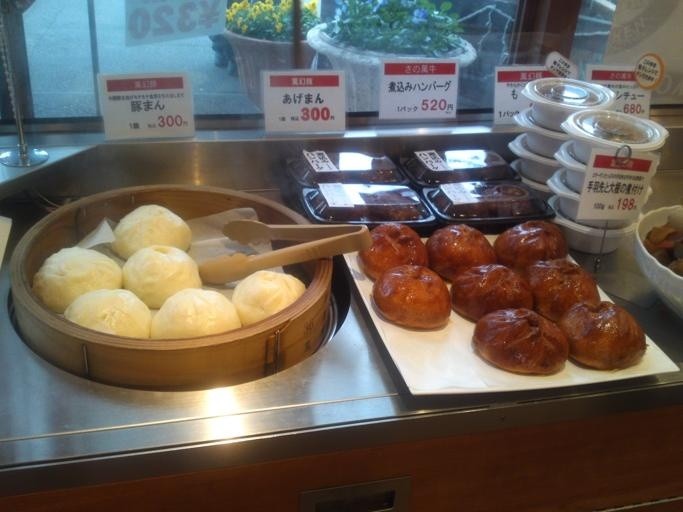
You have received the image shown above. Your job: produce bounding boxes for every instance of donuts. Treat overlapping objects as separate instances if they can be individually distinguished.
[298,152,540,220]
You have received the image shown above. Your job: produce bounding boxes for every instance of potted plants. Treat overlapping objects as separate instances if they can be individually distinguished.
[307,0,476,111]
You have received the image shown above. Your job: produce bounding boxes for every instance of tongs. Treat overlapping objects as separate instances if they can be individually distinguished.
[198,216,374,289]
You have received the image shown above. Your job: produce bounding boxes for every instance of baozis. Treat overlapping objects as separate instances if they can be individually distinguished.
[35,203,306,341]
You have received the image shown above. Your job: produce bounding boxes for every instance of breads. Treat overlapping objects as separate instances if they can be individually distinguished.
[357,222,645,375]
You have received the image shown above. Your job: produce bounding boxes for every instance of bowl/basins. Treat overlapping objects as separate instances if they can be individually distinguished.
[507,72,669,257]
[631,203,683,316]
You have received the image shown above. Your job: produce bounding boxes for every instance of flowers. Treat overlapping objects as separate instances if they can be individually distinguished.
[223,0,321,41]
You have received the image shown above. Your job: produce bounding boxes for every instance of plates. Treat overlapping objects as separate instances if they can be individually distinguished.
[337,228,681,395]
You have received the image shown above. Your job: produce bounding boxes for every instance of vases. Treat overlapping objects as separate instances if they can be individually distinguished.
[226,30,316,109]
